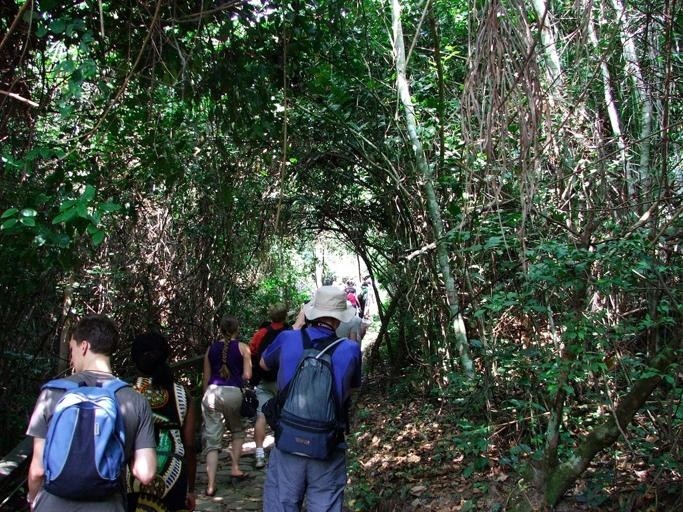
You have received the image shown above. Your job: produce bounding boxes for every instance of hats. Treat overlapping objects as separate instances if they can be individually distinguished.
[302,284,357,323]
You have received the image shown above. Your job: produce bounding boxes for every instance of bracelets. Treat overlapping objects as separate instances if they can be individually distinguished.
[26,494,33,503]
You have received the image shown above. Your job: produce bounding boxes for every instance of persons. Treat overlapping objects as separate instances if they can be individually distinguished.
[25,313,158,512]
[122,331,196,512]
[249,301,293,468]
[336,278,368,341]
[260,285,362,512]
[199,313,255,497]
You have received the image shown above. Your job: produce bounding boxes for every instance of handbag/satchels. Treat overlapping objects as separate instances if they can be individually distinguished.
[241,388,259,418]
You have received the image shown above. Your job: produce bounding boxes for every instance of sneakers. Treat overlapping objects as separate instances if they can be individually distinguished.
[255,456,266,468]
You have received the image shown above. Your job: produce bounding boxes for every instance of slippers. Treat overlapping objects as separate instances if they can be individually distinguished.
[228,470,249,480]
[206,484,218,497]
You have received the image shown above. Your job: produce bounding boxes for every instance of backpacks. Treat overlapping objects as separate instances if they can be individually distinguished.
[260,326,352,461]
[255,324,289,381]
[38,378,133,502]
